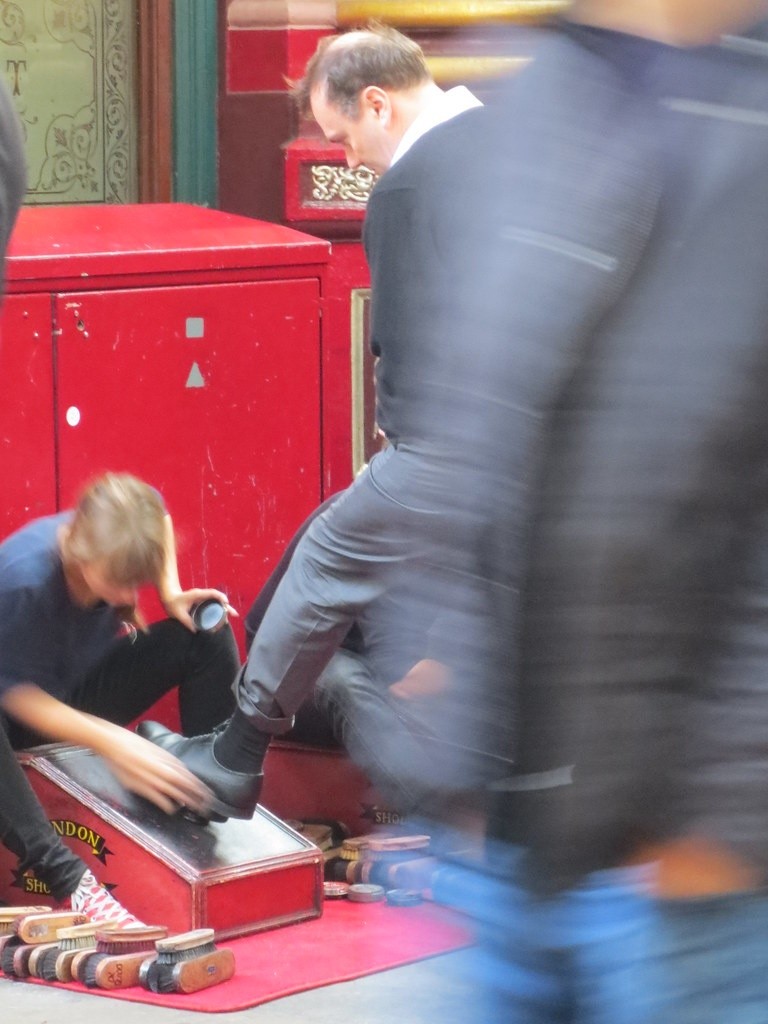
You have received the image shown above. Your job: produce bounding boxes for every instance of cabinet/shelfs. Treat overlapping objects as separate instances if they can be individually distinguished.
[0,200,387,741]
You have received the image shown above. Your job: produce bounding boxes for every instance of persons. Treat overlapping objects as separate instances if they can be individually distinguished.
[244,464,508,818]
[354,1,768,1022]
[1,469,246,936]
[131,22,508,820]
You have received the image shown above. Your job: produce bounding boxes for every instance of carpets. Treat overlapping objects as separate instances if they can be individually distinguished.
[0,897,481,1013]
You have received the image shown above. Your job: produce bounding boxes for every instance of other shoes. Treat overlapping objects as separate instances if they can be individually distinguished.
[68,874,144,932]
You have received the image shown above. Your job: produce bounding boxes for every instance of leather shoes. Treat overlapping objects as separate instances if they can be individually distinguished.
[134,719,263,819]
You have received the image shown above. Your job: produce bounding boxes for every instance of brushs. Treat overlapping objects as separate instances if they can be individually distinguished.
[316,832,437,888]
[0,905,237,995]
